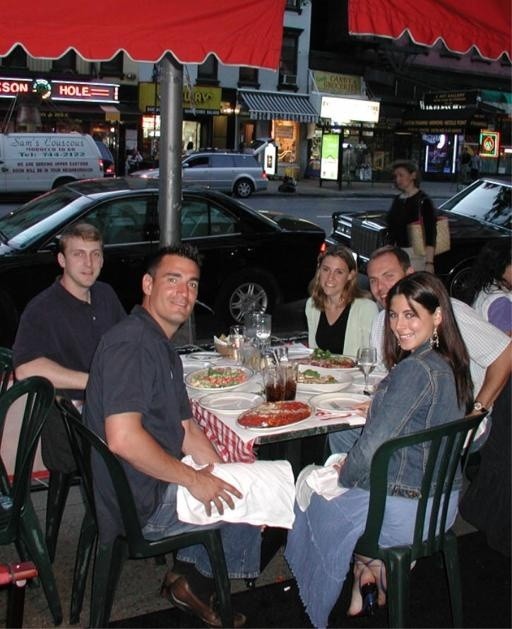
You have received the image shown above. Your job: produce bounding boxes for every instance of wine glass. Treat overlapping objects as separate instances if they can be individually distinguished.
[230,313,271,369]
[356,345,377,394]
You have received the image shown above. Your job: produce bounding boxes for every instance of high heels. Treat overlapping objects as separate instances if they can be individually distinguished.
[345,559,417,617]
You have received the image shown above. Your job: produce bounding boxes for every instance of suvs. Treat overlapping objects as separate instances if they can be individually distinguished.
[126,147,271,202]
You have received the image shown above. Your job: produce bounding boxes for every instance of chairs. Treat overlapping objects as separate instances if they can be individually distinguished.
[28,382,148,567]
[0,374,67,628]
[354,406,488,629]
[56,396,234,629]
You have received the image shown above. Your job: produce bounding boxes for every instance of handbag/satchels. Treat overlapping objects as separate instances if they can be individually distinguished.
[406,216,451,257]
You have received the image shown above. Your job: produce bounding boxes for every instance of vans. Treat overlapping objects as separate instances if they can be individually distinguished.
[0,130,117,198]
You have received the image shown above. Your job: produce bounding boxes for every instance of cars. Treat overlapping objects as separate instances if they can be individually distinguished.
[0,178,329,332]
[332,177,509,299]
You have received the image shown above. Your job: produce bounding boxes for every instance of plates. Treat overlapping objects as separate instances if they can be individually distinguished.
[236,401,312,432]
[185,365,253,392]
[307,355,360,372]
[297,364,354,394]
[308,393,372,413]
[199,392,264,414]
[288,347,314,360]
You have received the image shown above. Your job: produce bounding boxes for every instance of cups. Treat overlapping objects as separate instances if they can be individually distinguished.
[262,361,298,401]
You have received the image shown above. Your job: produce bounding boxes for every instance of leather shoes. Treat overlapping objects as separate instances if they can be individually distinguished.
[161,572,246,627]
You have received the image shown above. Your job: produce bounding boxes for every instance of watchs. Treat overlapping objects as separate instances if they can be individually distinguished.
[472,400,489,415]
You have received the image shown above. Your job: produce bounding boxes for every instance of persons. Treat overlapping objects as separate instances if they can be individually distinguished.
[81,242,264,629]
[283,270,475,618]
[13,222,127,478]
[472,238,512,338]
[365,244,511,455]
[305,246,379,362]
[388,159,438,271]
